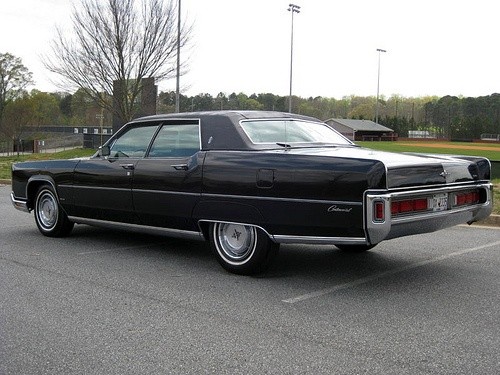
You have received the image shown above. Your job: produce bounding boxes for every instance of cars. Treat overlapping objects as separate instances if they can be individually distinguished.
[11,109,495,276]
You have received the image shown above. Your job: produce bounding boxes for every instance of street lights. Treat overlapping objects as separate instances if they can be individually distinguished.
[286,3,301,113]
[376,49,387,122]
